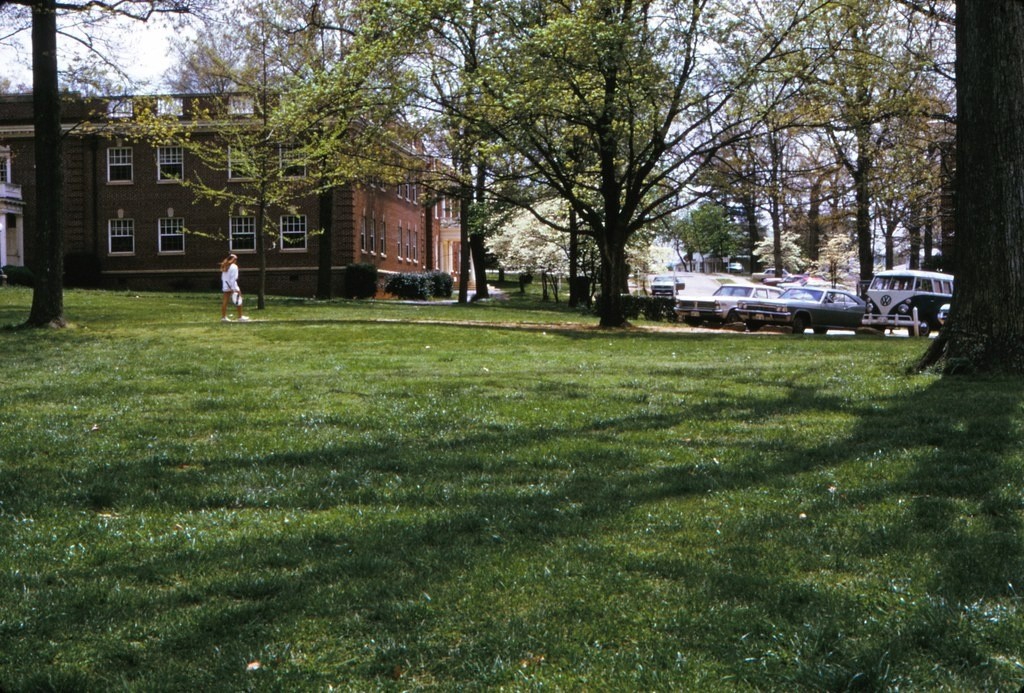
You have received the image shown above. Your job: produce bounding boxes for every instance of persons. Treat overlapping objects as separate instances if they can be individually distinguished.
[222,254,248,322]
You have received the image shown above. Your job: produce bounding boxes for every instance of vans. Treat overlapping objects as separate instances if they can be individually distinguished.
[861,268,954,337]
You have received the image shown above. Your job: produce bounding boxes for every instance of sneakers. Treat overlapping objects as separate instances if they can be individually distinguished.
[221,316,231,322]
[238,316,249,321]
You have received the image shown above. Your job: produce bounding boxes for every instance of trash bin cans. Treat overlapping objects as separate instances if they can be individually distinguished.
[567,276,590,306]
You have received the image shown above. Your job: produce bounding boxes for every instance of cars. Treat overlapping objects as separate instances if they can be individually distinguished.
[667,267,858,309]
[726,262,744,274]
[673,284,786,329]
[734,285,866,334]
[650,274,677,296]
